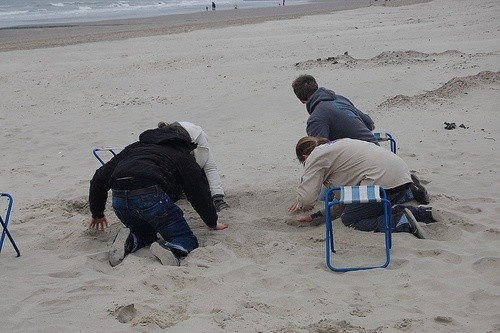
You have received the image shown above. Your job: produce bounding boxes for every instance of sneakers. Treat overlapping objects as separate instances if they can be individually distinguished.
[402,208,426,241]
[421,206,439,223]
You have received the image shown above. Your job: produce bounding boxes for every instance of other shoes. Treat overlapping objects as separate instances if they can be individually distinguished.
[409,182,429,206]
[212,196,231,213]
[411,173,420,184]
[149,242,180,267]
[108,227,131,267]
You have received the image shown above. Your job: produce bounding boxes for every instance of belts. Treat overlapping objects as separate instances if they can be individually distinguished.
[112,185,160,197]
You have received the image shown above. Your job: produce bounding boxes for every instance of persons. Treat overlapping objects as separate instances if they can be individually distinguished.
[289,135,438,239]
[155,121,230,212]
[89,121,227,267]
[292,74,430,206]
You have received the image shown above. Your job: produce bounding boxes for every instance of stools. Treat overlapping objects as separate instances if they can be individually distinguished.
[324,186,392,272]
[374,132,397,154]
